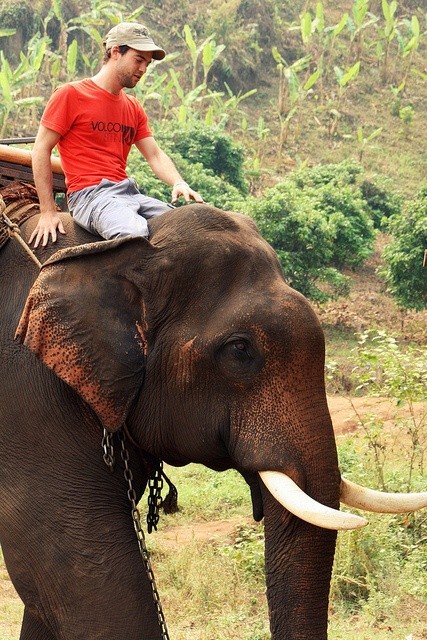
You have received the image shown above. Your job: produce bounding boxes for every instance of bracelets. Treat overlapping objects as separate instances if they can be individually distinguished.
[173,182,189,187]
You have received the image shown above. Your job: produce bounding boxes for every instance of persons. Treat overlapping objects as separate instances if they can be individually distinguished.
[27,23,204,249]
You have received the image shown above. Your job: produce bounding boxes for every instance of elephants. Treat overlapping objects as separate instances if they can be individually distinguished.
[0,180,426,640]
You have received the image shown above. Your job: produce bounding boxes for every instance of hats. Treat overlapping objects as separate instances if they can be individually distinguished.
[102,22,165,60]
